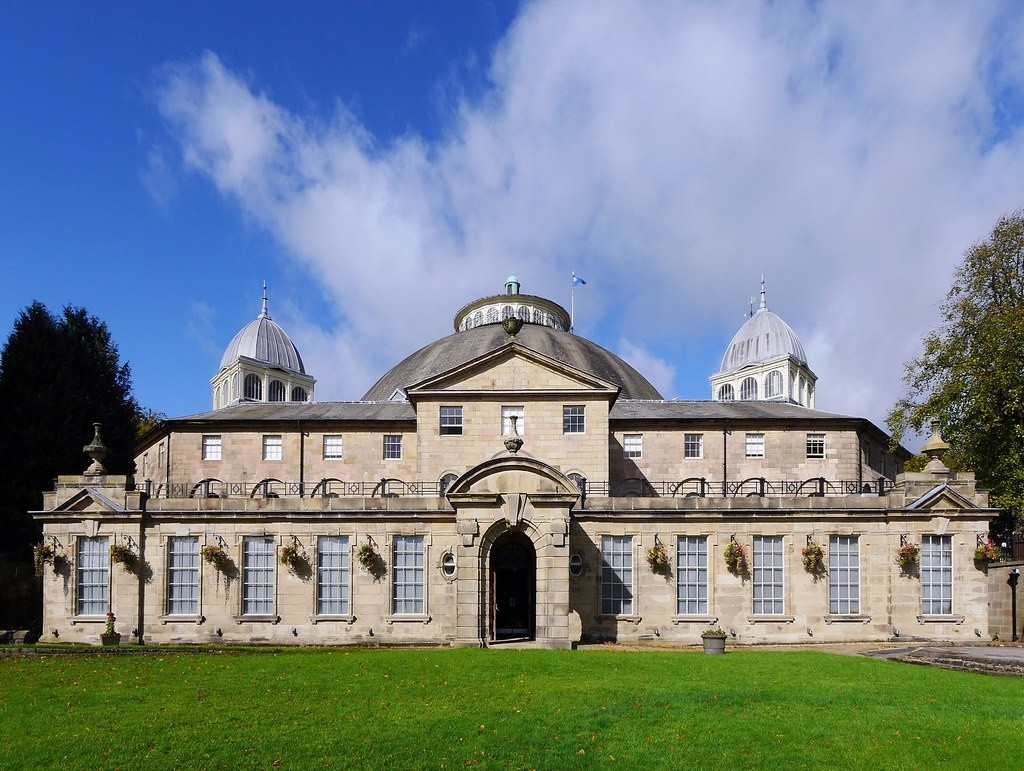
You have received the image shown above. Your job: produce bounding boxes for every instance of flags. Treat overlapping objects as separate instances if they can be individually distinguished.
[572,275,587,285]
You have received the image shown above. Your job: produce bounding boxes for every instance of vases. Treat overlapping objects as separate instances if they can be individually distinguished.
[101,632,122,646]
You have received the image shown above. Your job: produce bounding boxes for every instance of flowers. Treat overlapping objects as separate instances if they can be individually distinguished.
[896,542,920,567]
[103,612,116,634]
[645,542,669,575]
[111,543,134,564]
[724,540,750,576]
[974,538,1003,561]
[279,542,299,570]
[30,541,58,578]
[801,540,823,570]
[203,544,227,570]
[357,544,375,566]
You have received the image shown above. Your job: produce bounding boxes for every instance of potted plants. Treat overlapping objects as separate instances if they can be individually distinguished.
[701,626,727,655]
